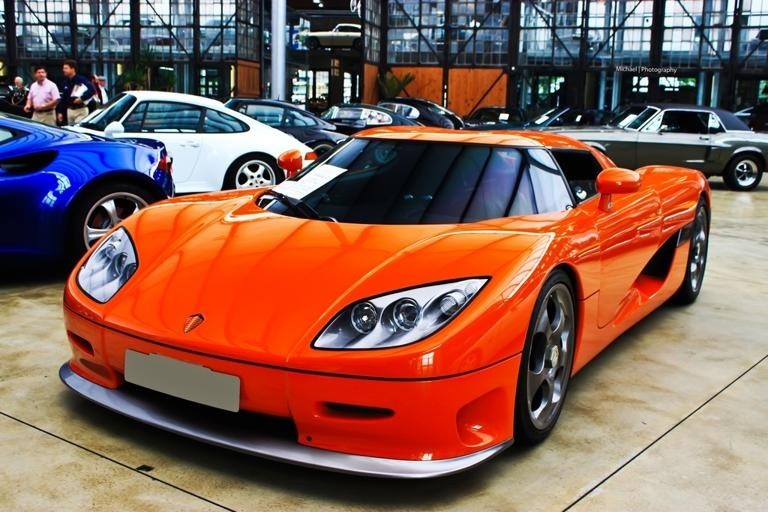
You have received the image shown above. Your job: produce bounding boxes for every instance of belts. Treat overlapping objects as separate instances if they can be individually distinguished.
[34,108,53,113]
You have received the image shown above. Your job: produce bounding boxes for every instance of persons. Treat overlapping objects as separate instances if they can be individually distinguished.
[94,75,109,105]
[22,65,61,128]
[55,58,98,126]
[5,74,29,105]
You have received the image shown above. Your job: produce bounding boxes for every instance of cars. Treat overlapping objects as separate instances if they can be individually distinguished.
[224,97,465,159]
[0,112,176,253]
[297,23,362,50]
[60,90,318,195]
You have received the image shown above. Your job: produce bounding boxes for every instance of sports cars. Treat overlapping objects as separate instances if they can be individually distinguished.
[59,126,711,478]
